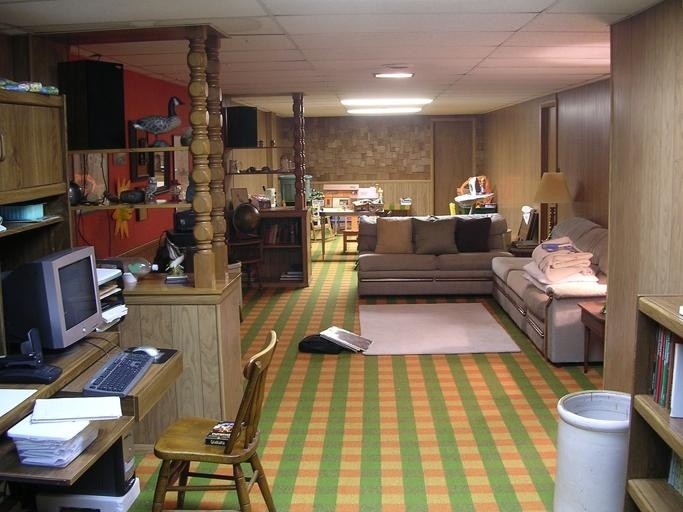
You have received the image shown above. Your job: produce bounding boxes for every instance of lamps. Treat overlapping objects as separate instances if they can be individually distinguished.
[533,171,571,243]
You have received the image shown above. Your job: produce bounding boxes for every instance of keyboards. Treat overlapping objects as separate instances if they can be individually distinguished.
[82,352,154,398]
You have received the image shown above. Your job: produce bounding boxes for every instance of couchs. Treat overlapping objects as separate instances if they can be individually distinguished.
[356,212,515,297]
[491,217,607,364]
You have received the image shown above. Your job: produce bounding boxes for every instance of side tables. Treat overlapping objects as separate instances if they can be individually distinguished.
[507,240,539,256]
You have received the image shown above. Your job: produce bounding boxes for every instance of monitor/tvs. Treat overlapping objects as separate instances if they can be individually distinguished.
[0,246,103,359]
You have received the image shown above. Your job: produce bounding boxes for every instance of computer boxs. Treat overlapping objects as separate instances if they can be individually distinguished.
[37,426,136,496]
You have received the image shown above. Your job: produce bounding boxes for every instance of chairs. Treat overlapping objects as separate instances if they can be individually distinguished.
[342,205,369,251]
[151,329,280,511]
[225,203,264,293]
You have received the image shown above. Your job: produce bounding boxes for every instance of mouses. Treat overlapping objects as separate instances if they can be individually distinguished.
[133,346,159,357]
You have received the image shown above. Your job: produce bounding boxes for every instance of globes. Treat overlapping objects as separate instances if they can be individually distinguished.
[232,198,262,239]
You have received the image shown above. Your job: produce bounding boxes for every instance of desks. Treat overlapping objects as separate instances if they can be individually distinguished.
[318,208,354,256]
[0,330,184,512]
[576,302,606,375]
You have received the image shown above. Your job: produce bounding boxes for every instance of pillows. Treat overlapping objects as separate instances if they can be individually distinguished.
[375,216,491,256]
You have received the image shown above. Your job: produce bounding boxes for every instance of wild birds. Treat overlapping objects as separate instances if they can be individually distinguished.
[129,96,185,140]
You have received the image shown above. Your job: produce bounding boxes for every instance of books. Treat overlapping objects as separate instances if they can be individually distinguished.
[81,268,128,333]
[279,266,304,282]
[262,218,300,245]
[666,450,682,497]
[642,327,682,417]
[540,243,579,255]
[205,420,246,448]
[319,326,372,353]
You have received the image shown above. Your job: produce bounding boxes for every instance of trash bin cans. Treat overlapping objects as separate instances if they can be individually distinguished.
[552,389,632,512]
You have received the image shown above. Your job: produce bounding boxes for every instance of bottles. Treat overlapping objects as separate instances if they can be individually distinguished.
[96,257,158,282]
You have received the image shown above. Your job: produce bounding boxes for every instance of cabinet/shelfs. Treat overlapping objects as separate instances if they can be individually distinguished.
[168,90,310,291]
[0,92,67,247]
[622,293,681,511]
[28,22,243,453]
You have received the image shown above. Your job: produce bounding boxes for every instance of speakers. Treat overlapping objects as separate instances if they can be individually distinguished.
[56,60,125,151]
[227,106,256,148]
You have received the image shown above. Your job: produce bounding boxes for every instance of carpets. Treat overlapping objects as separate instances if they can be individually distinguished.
[356,302,521,357]
